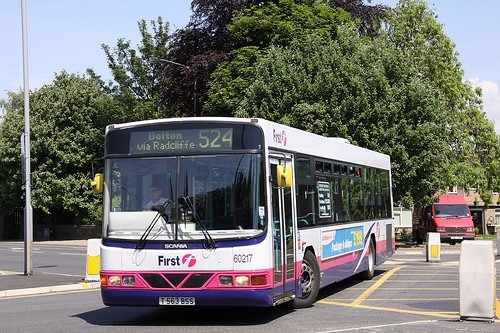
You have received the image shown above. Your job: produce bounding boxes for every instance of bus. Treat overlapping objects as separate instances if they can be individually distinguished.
[89,115,396,313]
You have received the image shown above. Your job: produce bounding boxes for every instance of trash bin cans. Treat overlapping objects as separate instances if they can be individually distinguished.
[41,226,55,240]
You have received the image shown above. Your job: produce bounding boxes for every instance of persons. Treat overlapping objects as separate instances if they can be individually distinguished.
[142,184,172,221]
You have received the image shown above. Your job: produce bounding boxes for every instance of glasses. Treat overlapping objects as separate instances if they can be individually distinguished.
[149,189,160,193]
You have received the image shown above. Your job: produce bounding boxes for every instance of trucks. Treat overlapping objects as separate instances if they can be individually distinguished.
[411,194,476,243]
